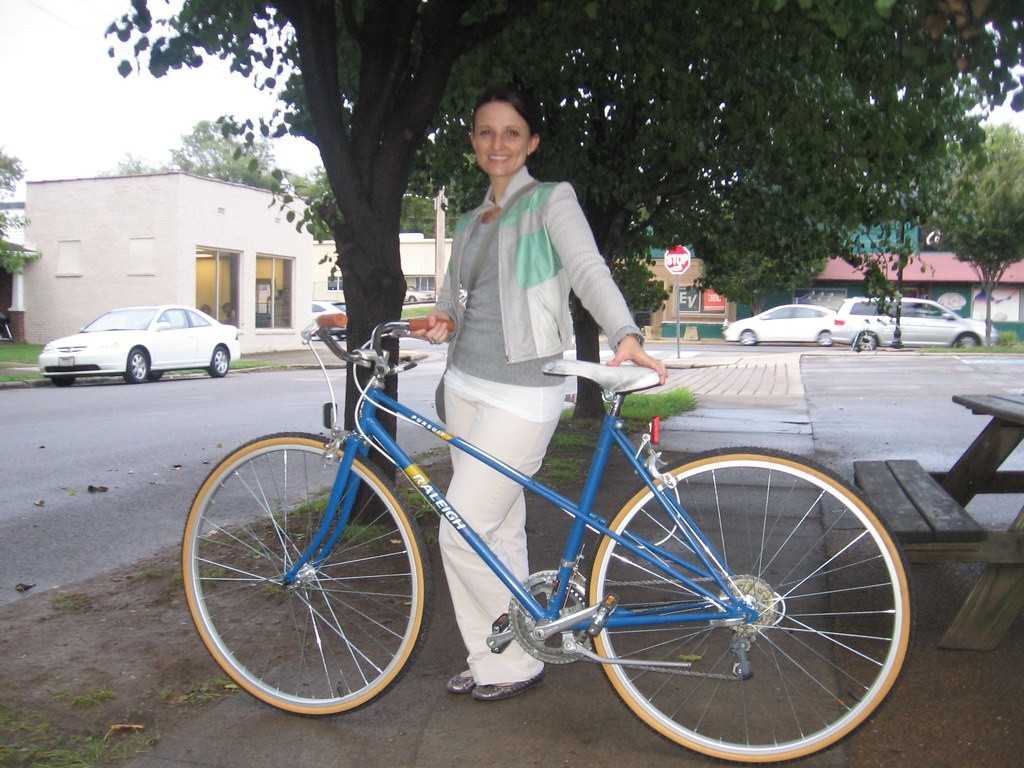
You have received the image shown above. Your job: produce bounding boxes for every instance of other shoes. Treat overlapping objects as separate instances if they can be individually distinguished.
[446,668,476,691]
[471,661,545,701]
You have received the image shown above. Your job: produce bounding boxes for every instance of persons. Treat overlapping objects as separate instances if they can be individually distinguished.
[411,86,668,701]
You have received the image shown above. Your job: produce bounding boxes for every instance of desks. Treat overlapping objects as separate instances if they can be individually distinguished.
[938,389,1024,653]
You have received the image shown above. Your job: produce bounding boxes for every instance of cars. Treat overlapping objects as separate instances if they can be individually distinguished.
[37,305,241,387]
[309,299,346,340]
[404,285,435,304]
[722,304,836,346]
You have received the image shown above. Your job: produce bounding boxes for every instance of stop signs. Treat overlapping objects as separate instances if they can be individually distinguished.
[663,245,691,274]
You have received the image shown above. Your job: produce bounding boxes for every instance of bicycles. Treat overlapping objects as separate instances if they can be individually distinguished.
[181,310,917,766]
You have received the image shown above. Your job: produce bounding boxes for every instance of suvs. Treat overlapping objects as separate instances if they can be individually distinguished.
[830,296,998,352]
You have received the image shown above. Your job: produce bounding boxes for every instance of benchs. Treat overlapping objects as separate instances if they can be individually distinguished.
[849,449,987,568]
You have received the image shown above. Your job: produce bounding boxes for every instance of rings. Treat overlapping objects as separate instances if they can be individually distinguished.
[429,338,436,344]
